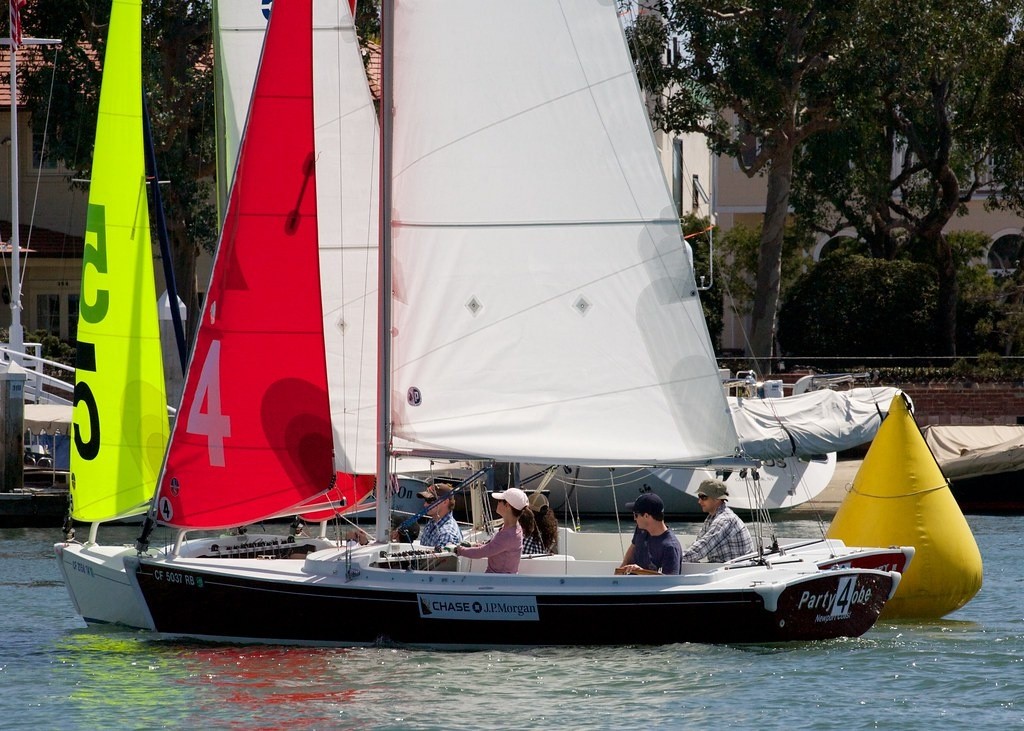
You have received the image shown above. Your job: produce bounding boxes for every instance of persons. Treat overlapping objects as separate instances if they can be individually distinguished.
[619,493,683,576]
[523,493,558,554]
[682,479,754,563]
[345,515,419,569]
[444,488,535,575]
[417,484,462,553]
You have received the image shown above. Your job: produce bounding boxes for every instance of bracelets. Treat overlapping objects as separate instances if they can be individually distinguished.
[360,541,369,546]
[347,539,357,542]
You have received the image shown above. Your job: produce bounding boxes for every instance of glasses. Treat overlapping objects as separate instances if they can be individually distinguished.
[497,499,504,503]
[634,513,644,519]
[423,497,436,504]
[698,494,708,501]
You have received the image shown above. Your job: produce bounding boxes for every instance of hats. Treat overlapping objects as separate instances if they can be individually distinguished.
[416,483,454,500]
[528,493,549,512]
[491,487,529,510]
[624,493,664,514]
[695,479,729,502]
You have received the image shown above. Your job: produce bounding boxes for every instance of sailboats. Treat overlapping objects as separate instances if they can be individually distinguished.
[0,1,179,526]
[55,0,1024,647]
